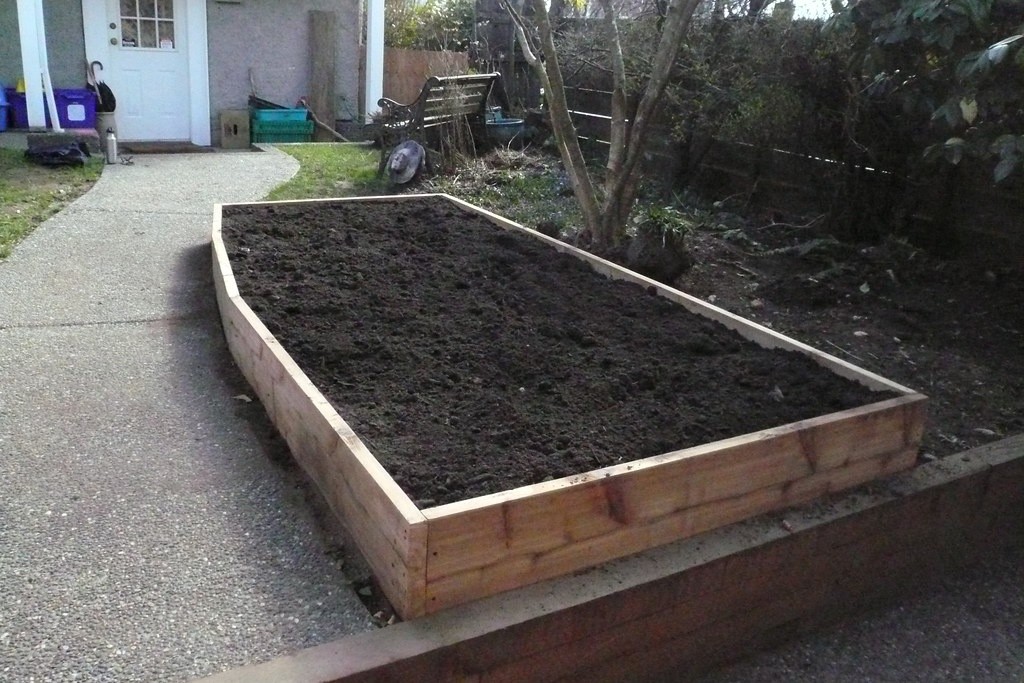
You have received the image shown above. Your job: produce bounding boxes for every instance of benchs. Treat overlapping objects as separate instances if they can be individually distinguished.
[377,70,500,178]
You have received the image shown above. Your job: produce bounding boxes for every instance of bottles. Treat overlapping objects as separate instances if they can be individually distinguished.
[106,127,117,164]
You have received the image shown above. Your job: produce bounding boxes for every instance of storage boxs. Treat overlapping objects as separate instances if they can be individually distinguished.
[0,84,98,129]
[253,106,315,142]
[220,107,251,149]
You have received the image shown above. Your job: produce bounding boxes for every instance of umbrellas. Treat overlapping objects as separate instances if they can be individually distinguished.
[82,61,116,112]
